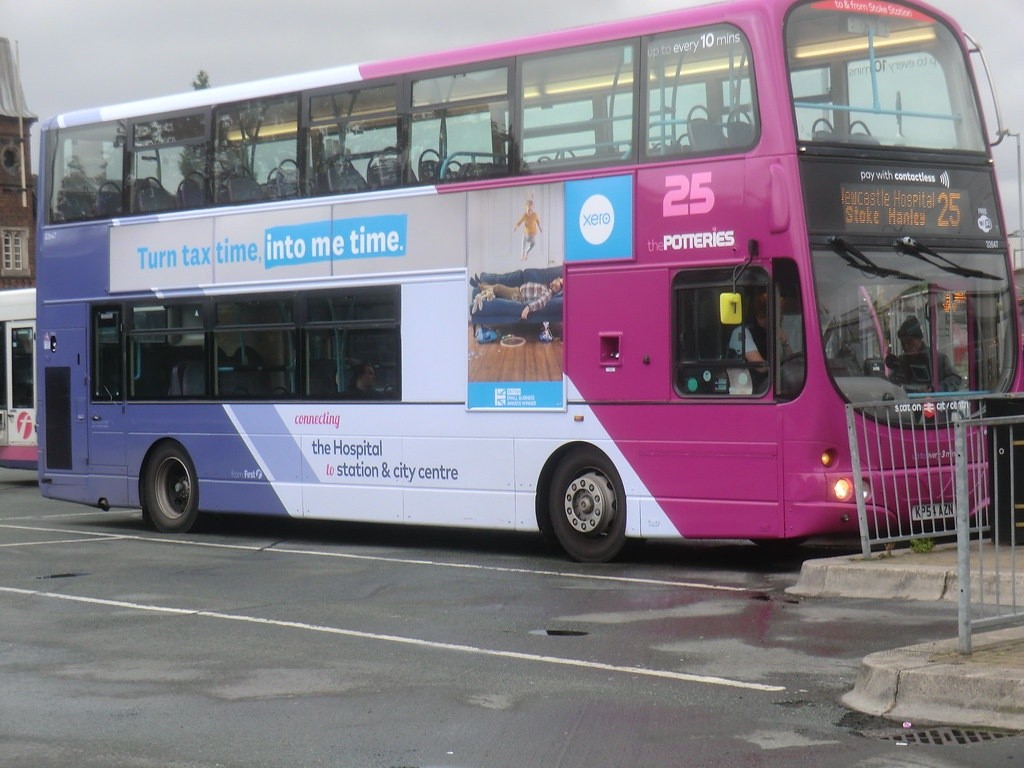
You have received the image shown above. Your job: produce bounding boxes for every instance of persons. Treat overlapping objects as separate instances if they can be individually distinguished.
[512,200,543,261]
[725,289,794,387]
[470,273,563,320]
[887,315,962,397]
[347,362,379,402]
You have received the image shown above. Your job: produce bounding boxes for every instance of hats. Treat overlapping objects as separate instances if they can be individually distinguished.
[897,314,923,338]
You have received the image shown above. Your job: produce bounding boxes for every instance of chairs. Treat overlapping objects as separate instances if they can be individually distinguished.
[55,99,880,222]
[99,343,399,399]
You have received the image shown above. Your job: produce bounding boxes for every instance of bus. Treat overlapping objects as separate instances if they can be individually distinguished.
[34,0,1023,567]
[1,289,37,470]
